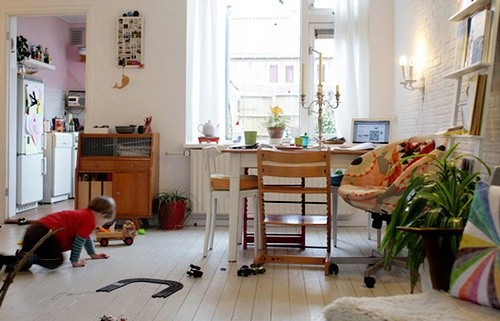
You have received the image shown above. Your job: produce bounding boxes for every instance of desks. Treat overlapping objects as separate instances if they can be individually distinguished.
[222,146,396,262]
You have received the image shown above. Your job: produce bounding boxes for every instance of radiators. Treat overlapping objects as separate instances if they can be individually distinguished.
[190,151,355,214]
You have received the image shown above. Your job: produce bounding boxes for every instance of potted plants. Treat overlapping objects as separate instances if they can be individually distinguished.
[151,183,197,230]
[376,140,492,293]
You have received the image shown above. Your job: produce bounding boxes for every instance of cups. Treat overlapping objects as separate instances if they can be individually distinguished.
[245,131,257,145]
[282,139,290,146]
[295,138,302,146]
[300,136,308,147]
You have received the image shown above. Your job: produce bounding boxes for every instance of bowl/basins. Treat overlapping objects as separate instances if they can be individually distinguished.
[89,125,112,134]
[116,126,135,134]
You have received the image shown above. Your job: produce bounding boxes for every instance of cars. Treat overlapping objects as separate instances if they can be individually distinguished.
[187,263,203,278]
[236,262,265,276]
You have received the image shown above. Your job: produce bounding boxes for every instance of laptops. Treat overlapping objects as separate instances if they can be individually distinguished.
[339,117,396,150]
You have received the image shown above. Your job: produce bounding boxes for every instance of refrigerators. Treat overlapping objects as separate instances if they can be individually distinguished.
[16,72,48,215]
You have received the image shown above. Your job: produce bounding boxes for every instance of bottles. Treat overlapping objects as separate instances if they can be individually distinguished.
[31,44,50,64]
[69,120,75,132]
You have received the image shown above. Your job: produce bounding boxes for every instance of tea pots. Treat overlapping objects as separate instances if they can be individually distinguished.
[198,120,220,137]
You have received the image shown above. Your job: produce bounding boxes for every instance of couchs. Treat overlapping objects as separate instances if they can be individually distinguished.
[323,185,500,321]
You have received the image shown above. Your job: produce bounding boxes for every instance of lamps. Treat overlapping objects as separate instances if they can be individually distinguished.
[398,56,422,91]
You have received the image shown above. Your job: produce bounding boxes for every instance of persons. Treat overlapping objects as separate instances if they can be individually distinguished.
[0,196,116,273]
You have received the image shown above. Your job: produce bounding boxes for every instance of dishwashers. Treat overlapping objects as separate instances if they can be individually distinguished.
[41,134,72,203]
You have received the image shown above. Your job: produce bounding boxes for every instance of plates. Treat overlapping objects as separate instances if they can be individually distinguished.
[322,140,345,144]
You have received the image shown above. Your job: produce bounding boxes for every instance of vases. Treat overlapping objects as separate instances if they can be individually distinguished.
[267,127,286,137]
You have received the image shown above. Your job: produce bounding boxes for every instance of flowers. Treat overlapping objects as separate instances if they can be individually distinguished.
[261,106,291,129]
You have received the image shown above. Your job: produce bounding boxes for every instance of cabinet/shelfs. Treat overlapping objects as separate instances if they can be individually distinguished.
[442,0,500,154]
[75,133,160,219]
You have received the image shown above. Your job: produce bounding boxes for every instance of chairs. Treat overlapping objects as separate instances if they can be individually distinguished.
[203,146,263,258]
[254,147,332,276]
[328,137,446,288]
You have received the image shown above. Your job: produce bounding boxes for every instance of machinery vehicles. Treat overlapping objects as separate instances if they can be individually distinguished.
[93,220,135,247]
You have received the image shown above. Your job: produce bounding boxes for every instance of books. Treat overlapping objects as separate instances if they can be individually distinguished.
[451,1,493,183]
